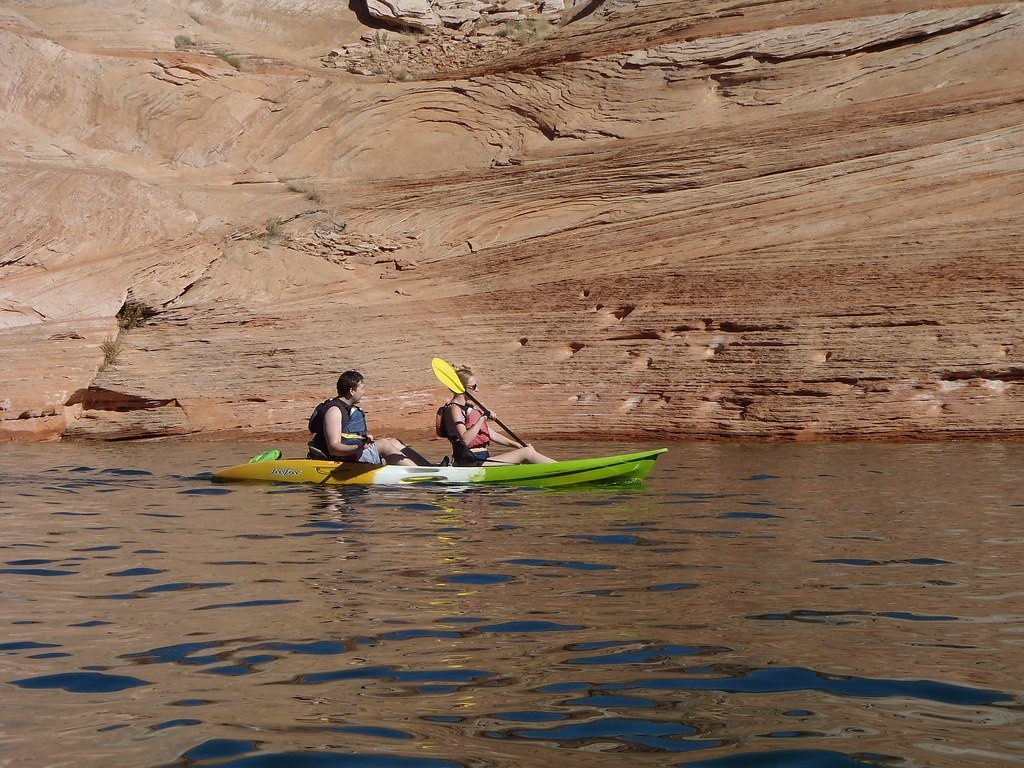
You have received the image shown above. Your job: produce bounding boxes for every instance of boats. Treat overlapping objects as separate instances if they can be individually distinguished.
[198,448,670,488]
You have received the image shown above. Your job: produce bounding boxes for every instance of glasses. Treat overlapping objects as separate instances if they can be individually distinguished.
[439,456,449,467]
[464,384,476,390]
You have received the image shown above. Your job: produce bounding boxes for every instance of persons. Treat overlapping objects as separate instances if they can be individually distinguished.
[444,369,558,466]
[319,370,449,468]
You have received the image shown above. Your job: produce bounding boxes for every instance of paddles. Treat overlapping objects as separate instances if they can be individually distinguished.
[341,432,373,442]
[432,358,528,448]
